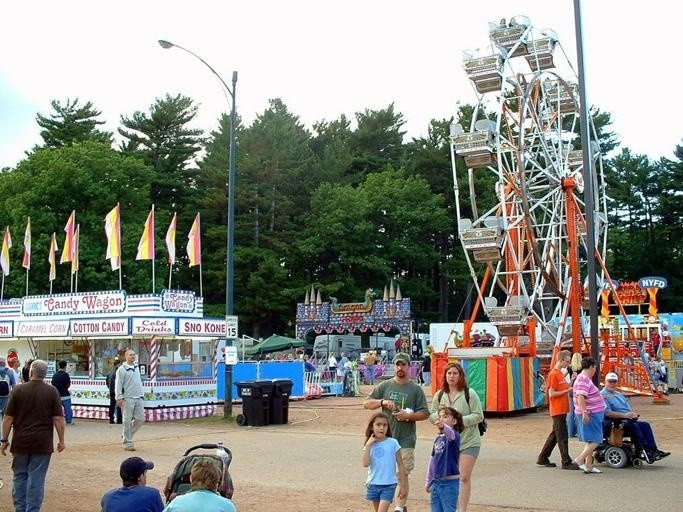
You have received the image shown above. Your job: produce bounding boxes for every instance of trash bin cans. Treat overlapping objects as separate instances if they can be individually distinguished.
[233,380,275,426]
[269,379,294,424]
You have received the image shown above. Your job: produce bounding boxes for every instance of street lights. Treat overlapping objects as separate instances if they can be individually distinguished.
[158,38,237,417]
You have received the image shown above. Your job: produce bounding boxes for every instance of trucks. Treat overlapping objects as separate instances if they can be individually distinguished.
[314,335,361,358]
[395,334,429,357]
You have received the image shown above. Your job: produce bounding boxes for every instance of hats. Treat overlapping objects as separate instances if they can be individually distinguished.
[391,352,410,365]
[606,372,618,381]
[120,457,154,481]
[0,358,7,363]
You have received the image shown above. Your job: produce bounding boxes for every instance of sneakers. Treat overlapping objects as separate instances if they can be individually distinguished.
[125,446,135,451]
[645,449,671,461]
[66,421,74,426]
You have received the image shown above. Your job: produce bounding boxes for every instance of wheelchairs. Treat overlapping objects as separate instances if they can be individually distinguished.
[596,413,657,468]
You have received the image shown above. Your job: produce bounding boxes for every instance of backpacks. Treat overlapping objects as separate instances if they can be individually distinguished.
[438,388,487,436]
[0,375,11,399]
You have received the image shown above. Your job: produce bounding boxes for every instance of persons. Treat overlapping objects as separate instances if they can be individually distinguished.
[538,340,671,475]
[106,360,123,424]
[496,17,508,30]
[0,355,76,511]
[509,16,522,28]
[366,353,429,511]
[101,457,166,511]
[480,329,489,340]
[161,458,236,512]
[425,409,461,512]
[115,350,146,451]
[364,413,408,511]
[264,339,434,386]
[432,364,484,512]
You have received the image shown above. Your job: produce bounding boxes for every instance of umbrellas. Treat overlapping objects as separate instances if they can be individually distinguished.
[245,334,306,360]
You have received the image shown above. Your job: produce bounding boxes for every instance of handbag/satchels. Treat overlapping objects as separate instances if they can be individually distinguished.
[607,427,624,447]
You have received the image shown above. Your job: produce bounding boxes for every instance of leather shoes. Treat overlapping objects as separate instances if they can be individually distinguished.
[562,462,579,470]
[544,461,556,468]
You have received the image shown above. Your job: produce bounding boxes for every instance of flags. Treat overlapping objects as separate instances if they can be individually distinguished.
[71,223,80,273]
[23,217,32,271]
[163,212,178,263]
[48,231,59,281]
[60,210,75,264]
[1,227,13,275]
[187,212,202,267]
[104,203,120,273]
[134,205,156,261]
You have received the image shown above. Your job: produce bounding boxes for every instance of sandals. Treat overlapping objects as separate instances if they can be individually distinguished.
[584,466,603,474]
[571,459,586,470]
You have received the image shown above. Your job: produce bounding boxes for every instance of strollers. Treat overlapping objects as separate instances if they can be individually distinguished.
[162,443,234,504]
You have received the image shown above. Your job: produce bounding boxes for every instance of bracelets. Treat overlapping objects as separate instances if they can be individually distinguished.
[381,399,385,406]
